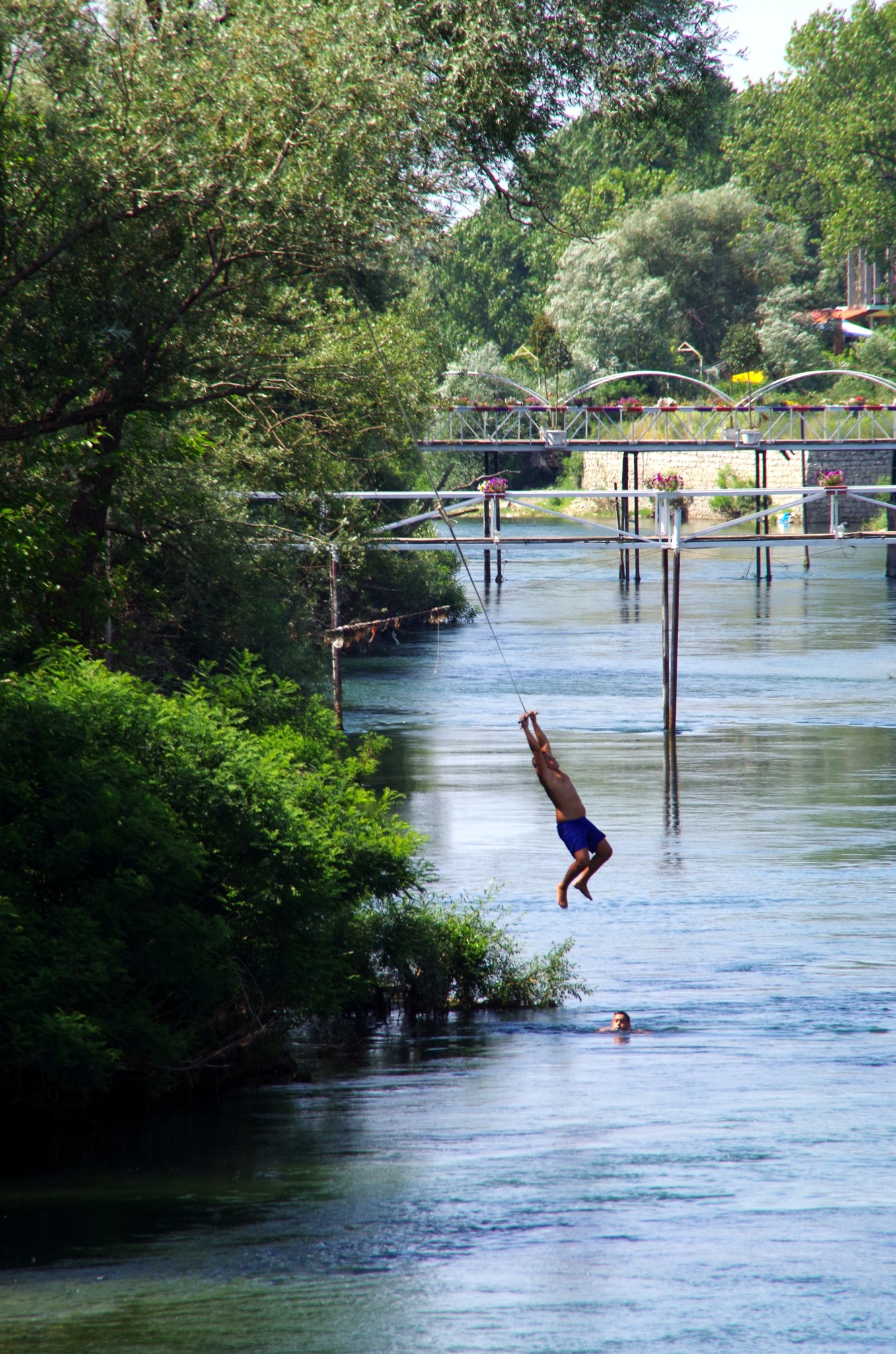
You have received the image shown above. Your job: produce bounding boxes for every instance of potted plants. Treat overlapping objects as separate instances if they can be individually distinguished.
[717,324,758,444]
[525,313,574,446]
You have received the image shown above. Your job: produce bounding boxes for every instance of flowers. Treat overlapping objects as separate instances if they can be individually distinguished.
[575,396,802,414]
[814,469,846,486]
[438,395,534,412]
[643,473,684,505]
[820,395,878,419]
[477,478,508,491]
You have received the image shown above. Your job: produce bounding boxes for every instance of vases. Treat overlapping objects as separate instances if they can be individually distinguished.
[484,490,505,498]
[825,486,847,495]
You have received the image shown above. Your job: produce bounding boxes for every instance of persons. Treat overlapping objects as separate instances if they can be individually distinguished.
[520,712,613,910]
[612,1011,630,1031]
